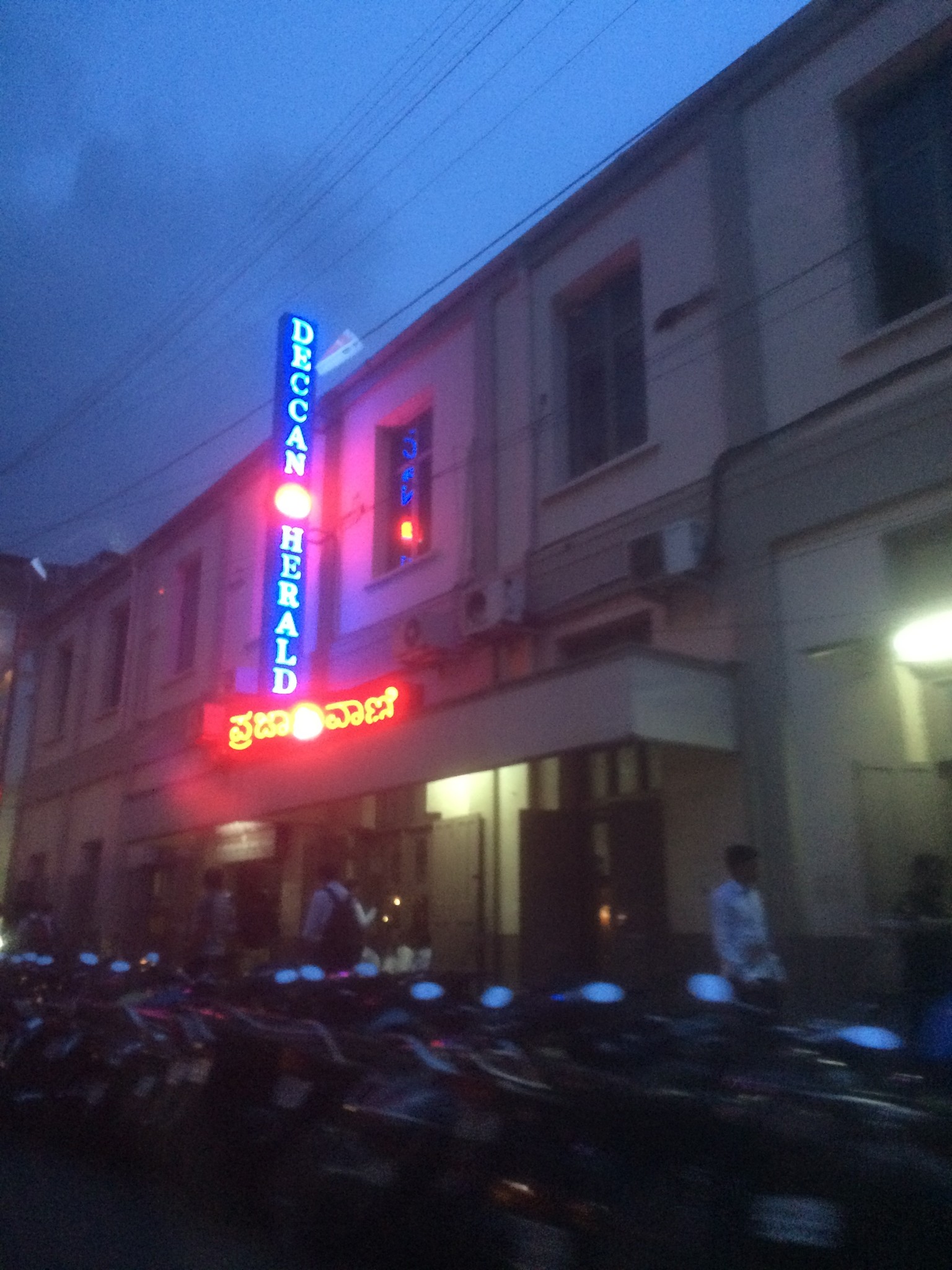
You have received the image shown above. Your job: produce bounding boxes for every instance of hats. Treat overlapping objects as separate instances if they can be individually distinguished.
[723,844,756,863]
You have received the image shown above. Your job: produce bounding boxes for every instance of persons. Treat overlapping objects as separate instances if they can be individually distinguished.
[880,856,952,1026]
[183,867,236,980]
[303,862,378,971]
[16,901,57,956]
[711,844,786,989]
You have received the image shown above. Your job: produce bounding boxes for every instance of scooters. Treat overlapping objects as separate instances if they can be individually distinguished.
[1,944,952,1269]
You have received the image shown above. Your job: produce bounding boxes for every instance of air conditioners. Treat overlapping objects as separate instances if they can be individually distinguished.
[628,520,700,585]
[458,577,514,640]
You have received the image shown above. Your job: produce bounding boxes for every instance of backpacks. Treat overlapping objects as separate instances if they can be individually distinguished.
[316,885,365,973]
